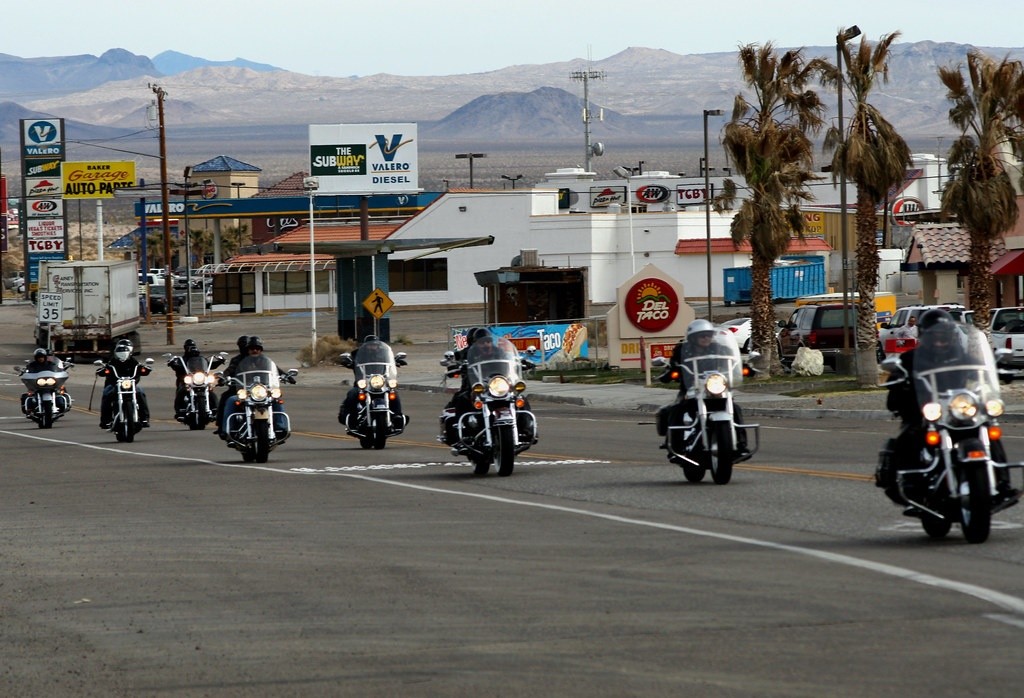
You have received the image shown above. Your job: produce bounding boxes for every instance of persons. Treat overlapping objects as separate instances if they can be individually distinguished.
[874,309,1023,514]
[21,348,68,418]
[338,335,410,426]
[96,339,152,429]
[655,320,752,456]
[167,339,227,420]
[212,335,296,444]
[440,327,539,453]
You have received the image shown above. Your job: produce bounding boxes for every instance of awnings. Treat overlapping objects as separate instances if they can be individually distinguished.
[989,250,1024,276]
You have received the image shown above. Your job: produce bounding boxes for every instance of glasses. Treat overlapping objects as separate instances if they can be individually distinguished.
[696,332,712,338]
[477,340,491,344]
[249,346,261,351]
[926,332,952,344]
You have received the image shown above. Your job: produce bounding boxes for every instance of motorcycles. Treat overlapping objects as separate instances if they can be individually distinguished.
[652,321,762,485]
[870,319,1023,546]
[436,334,538,478]
[162,351,229,431]
[337,340,408,450]
[92,357,155,443]
[14,357,75,429]
[212,353,299,465]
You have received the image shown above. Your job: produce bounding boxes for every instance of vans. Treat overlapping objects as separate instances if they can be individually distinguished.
[988,306,1024,384]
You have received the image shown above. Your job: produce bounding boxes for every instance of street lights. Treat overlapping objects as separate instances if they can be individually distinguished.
[455,153,486,189]
[231,182,246,247]
[834,25,862,352]
[302,176,319,367]
[701,108,726,323]
[182,190,203,318]
[501,174,523,189]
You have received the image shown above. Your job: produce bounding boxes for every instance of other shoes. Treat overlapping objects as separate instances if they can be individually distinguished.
[902,505,919,517]
[660,441,669,449]
[102,422,113,428]
[226,438,235,448]
[669,454,687,463]
[142,420,150,427]
[276,433,285,445]
[738,447,751,460]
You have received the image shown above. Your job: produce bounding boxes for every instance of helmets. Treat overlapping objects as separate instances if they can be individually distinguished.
[184,339,196,348]
[918,310,955,340]
[687,319,715,337]
[473,327,492,347]
[237,336,251,345]
[467,327,479,346]
[114,345,130,362]
[33,348,48,362]
[116,339,133,355]
[364,335,379,345]
[246,336,263,348]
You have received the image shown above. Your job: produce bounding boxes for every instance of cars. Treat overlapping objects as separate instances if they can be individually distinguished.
[3,270,37,304]
[718,316,754,354]
[138,264,214,315]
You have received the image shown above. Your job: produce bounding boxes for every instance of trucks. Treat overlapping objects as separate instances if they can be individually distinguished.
[31,258,141,365]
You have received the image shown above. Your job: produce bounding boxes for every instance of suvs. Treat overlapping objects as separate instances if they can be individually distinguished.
[875,302,977,366]
[774,298,861,373]
